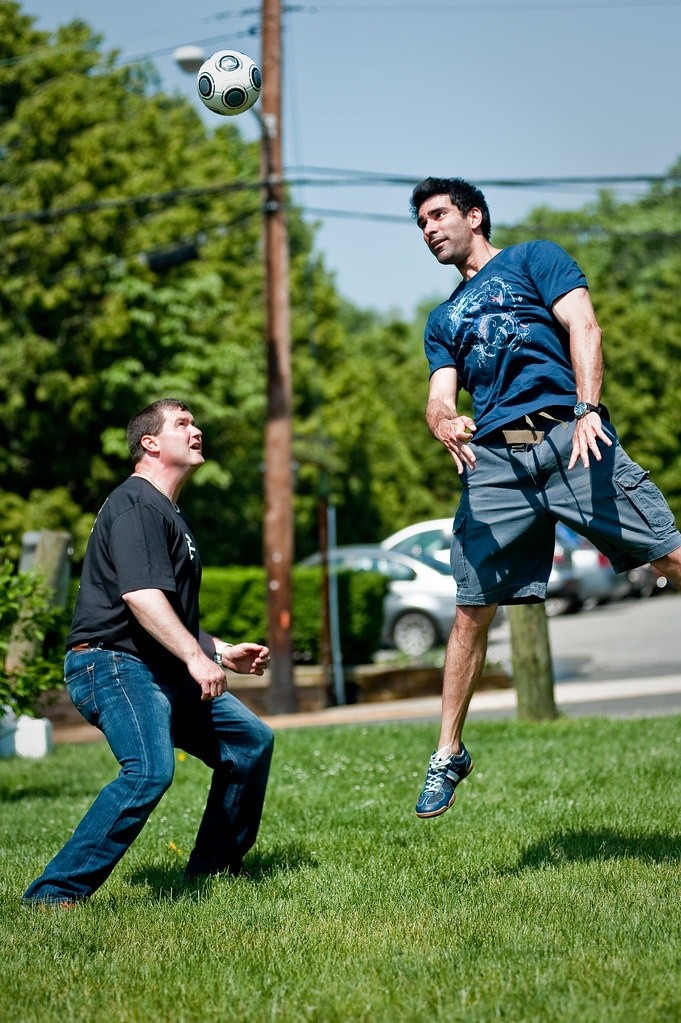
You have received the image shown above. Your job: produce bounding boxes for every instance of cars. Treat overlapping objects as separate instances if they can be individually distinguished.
[572,530,673,606]
[387,518,578,619]
[297,545,514,648]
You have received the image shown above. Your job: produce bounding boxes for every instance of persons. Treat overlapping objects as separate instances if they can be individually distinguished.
[409,175,681,819]
[20,398,277,916]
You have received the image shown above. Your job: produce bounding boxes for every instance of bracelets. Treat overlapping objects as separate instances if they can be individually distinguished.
[213,642,233,669]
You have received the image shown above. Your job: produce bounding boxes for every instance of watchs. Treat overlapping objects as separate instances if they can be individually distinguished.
[574,402,602,420]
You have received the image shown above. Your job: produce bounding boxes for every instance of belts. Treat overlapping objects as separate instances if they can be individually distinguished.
[87,641,140,656]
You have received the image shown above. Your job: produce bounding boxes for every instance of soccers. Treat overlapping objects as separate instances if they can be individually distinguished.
[196,50,261,117]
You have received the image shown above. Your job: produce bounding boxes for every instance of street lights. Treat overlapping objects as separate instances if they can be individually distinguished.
[177,45,305,713]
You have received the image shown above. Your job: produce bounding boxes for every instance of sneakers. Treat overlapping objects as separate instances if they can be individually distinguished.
[416,741,474,818]
